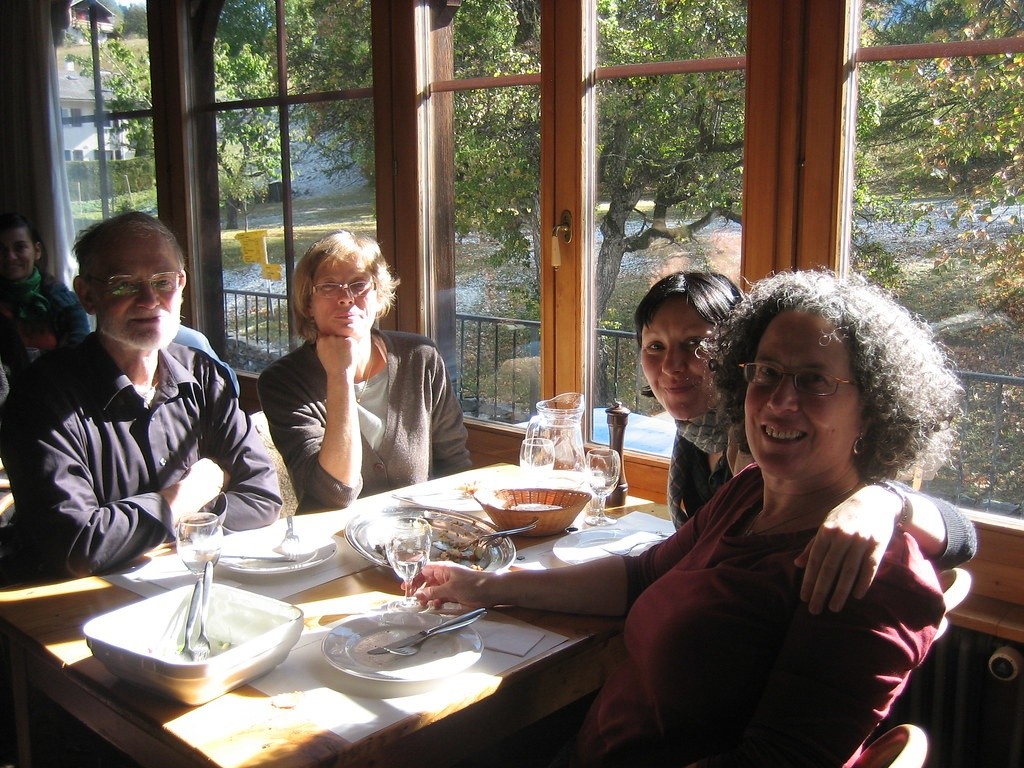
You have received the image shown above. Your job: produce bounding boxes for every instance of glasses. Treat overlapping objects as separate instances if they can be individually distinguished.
[87,271,184,297]
[312,280,377,299]
[739,363,859,396]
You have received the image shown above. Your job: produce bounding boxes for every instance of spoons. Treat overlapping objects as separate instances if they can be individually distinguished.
[431,523,537,558]
[180,579,203,663]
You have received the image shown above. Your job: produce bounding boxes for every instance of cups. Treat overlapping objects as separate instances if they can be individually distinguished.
[175,512,221,582]
[518,438,555,490]
[25,347,40,363]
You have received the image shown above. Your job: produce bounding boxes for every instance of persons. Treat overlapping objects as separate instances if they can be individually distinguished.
[634,269,977,614]
[0,212,89,363]
[256,228,471,514]
[0,213,284,577]
[173,325,240,397]
[401,269,967,768]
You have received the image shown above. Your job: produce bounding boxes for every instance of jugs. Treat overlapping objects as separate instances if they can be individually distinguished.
[524,390,586,490]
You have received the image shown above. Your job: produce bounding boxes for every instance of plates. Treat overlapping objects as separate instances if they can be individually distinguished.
[554,529,650,566]
[412,491,483,512]
[219,529,337,575]
[321,612,484,683]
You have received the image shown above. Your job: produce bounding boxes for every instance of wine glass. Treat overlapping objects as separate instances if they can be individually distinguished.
[384,517,432,614]
[585,448,622,528]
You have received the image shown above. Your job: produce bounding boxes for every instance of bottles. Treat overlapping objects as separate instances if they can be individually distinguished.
[605,401,629,507]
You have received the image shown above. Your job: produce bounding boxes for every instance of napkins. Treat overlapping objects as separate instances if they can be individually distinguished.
[470,621,546,657]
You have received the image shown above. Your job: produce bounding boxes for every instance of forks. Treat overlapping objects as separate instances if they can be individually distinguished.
[380,612,487,655]
[192,561,214,661]
[285,514,300,558]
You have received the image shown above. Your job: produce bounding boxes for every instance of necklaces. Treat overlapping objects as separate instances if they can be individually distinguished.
[356,350,375,403]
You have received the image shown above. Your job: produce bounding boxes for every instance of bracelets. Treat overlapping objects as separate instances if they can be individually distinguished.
[878,481,908,526]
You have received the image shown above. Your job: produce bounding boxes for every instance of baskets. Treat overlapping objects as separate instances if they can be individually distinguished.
[475,486,593,537]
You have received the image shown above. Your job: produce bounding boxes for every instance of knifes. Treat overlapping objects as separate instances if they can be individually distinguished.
[366,608,487,656]
[219,555,297,562]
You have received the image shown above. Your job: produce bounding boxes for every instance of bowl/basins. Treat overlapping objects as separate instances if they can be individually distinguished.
[81,583,304,707]
[343,505,516,576]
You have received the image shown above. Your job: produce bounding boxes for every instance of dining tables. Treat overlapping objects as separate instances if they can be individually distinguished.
[0,460,676,767]
